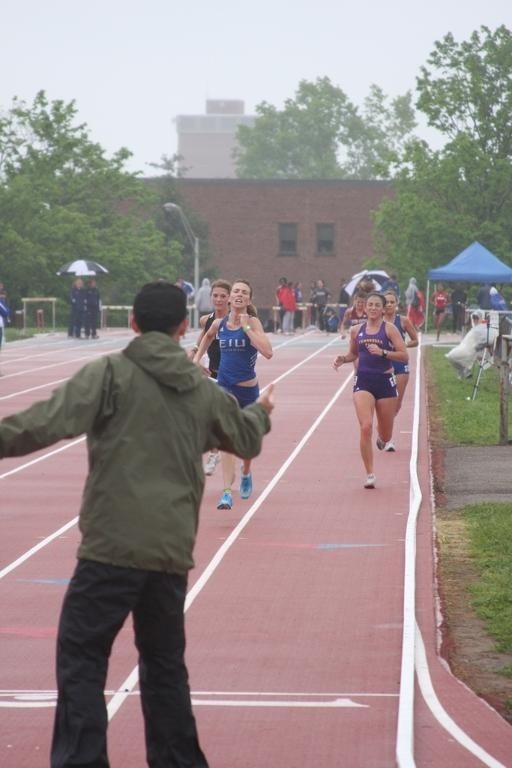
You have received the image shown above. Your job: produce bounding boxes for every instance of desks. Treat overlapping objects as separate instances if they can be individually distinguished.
[272,302,313,333]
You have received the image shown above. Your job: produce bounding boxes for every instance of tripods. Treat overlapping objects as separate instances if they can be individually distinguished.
[472,311,499,400]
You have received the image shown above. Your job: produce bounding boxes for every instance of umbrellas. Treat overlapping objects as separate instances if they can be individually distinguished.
[345,269,390,295]
[55,257,110,277]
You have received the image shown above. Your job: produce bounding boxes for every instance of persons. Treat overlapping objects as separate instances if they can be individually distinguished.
[68,277,87,340]
[86,280,101,340]
[184,278,233,475]
[276,275,508,343]
[173,275,195,301]
[332,293,409,489]
[383,289,419,452]
[192,278,274,510]
[0,292,13,351]
[195,277,216,315]
[0,279,274,767]
[339,292,369,387]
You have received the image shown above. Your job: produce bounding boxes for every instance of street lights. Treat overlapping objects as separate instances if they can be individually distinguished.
[163,202,200,327]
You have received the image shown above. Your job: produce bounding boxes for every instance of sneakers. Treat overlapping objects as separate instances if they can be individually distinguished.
[385,442,395,451]
[363,473,377,487]
[238,465,253,499]
[216,490,233,509]
[203,452,221,476]
[377,436,386,449]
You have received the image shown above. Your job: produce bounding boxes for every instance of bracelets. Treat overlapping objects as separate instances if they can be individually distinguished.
[342,355,346,363]
[192,345,199,353]
[382,349,388,359]
[242,324,251,333]
